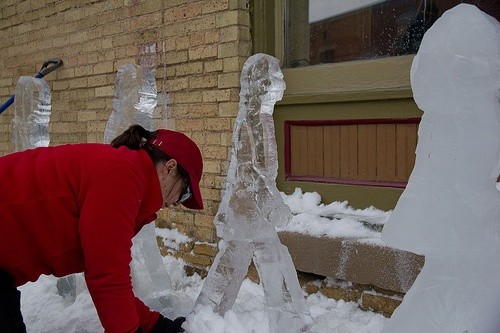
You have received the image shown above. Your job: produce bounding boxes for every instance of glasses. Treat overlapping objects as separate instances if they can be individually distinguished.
[178,164,193,203]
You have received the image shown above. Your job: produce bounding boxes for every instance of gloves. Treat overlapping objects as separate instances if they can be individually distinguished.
[149,313,186,333]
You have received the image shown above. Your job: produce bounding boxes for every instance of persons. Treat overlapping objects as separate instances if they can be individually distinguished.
[0,123,206,333]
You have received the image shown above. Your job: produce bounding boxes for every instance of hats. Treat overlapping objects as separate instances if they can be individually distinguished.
[146,128,205,211]
